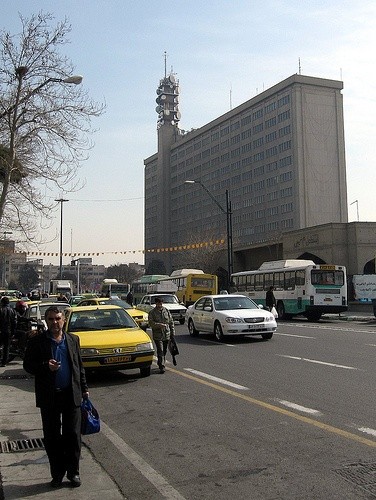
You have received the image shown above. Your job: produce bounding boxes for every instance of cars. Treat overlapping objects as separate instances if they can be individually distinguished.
[0,288,102,332]
[76,295,151,332]
[61,304,155,377]
[184,294,278,343]
[135,293,187,325]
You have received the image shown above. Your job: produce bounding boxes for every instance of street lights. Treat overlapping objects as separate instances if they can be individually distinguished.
[184,180,234,286]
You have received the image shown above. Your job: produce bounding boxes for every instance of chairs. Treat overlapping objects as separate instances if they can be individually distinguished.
[104,317,111,323]
[74,319,85,326]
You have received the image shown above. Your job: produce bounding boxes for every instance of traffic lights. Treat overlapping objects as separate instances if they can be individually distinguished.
[71,260,75,266]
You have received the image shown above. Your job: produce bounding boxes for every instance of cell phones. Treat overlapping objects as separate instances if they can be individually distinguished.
[53,362,61,365]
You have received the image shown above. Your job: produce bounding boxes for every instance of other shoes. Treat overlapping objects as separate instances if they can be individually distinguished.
[70,475,80,487]
[160,366,165,373]
[3,363,8,366]
[50,479,62,488]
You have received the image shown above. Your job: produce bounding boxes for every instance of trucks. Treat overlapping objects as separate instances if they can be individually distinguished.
[48,279,73,303]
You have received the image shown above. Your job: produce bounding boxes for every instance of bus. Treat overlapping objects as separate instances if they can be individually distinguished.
[157,268,218,309]
[230,257,348,323]
[99,278,130,303]
[130,274,170,306]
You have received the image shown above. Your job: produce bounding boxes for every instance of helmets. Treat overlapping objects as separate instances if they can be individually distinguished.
[15,301,26,312]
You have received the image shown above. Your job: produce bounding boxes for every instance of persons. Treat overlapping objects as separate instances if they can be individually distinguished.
[266,286,276,312]
[0,290,111,303]
[12,301,37,353]
[127,291,133,306]
[0,297,17,366]
[23,306,89,488]
[148,297,175,373]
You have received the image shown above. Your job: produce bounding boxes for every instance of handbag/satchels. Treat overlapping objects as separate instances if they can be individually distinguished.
[271,306,278,318]
[168,339,180,356]
[81,395,100,435]
